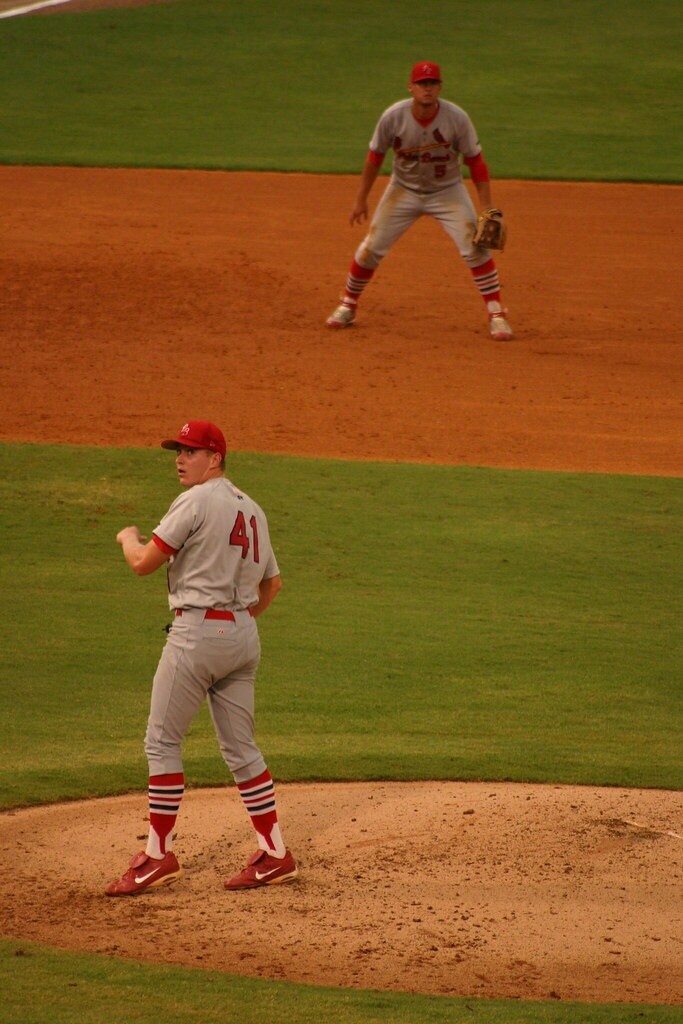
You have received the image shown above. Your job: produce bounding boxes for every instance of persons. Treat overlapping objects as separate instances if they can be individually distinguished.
[103,421,297,896]
[323,62,514,339]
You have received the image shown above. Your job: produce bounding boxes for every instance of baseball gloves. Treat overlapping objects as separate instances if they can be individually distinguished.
[471,207,513,258]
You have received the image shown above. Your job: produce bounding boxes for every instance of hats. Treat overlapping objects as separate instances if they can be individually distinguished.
[160,418,226,460]
[410,61,442,83]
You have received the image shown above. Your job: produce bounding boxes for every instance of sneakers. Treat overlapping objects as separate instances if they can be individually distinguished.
[224,849,300,890]
[487,312,513,341]
[326,301,357,329]
[104,850,184,897]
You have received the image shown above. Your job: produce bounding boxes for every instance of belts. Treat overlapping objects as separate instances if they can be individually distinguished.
[177,606,254,621]
